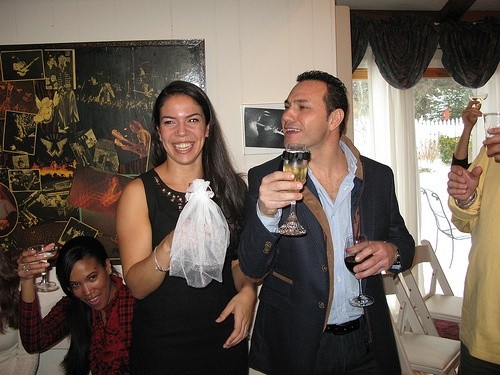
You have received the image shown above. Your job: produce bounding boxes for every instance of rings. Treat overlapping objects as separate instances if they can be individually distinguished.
[21,265,26,271]
[22,257,26,263]
[244,330,249,335]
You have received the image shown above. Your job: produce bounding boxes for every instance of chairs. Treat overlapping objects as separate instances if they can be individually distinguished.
[380,239,463,375]
[419,186,471,268]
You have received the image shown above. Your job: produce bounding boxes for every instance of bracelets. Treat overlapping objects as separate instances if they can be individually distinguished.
[455,192,477,209]
[153,246,171,272]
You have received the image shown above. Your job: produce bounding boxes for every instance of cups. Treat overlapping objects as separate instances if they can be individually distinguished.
[483,111,500,142]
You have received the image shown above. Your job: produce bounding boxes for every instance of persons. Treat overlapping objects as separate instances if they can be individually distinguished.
[16,235,139,375]
[0,50,152,241]
[450,100,483,170]
[236,71,415,375]
[116,81,259,375]
[447,99,500,375]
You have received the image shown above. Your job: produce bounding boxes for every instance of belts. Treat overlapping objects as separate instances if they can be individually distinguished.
[324,315,367,335]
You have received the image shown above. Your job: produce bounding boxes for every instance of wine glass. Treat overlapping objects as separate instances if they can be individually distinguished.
[28,244,59,293]
[469,89,489,116]
[343,234,375,308]
[280,144,309,236]
